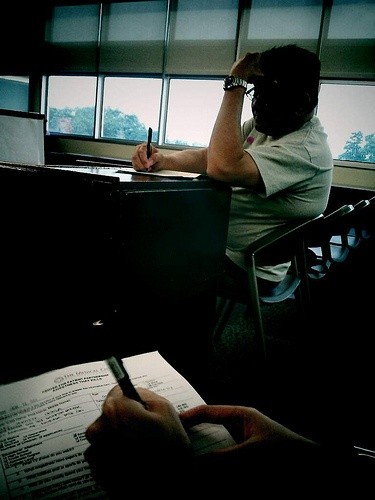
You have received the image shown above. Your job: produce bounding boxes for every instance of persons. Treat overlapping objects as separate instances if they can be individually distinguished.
[132,44,334,302]
[82,385,375,500]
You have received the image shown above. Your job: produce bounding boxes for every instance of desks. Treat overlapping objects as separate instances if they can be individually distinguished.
[0,161,233,360]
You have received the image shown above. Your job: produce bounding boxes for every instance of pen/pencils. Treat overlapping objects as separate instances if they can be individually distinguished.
[146,127,152,173]
[92,321,142,402]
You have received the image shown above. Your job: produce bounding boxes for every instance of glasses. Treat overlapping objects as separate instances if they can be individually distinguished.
[246,87,260,102]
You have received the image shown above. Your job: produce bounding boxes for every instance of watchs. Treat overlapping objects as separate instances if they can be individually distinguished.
[222,75,248,90]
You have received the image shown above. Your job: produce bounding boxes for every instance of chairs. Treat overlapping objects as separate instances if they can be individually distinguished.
[241,197,375,357]
